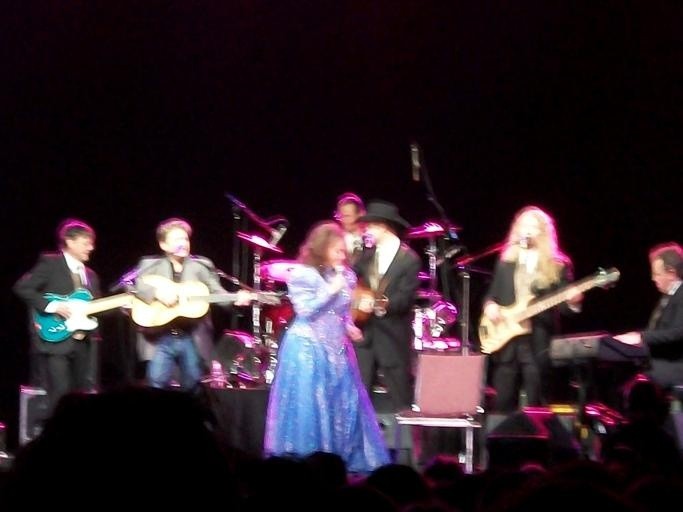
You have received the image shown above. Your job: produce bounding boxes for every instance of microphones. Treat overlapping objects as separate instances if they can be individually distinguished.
[412,147,423,181]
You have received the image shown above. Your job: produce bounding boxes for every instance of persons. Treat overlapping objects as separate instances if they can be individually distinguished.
[13,221,102,411]
[0,369,682,511]
[117,219,250,392]
[332,193,367,256]
[476,206,585,417]
[261,222,393,476]
[332,204,424,412]
[610,244,683,416]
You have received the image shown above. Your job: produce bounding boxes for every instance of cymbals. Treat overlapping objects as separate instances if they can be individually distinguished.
[237,230,283,255]
[408,223,459,240]
[257,262,302,281]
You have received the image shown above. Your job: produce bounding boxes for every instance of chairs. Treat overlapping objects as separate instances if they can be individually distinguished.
[394,355,486,474]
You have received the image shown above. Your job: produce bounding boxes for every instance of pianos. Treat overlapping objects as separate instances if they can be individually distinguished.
[549,332,649,369]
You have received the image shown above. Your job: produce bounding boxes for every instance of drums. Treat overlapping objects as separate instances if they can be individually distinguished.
[214,334,277,386]
[411,337,463,380]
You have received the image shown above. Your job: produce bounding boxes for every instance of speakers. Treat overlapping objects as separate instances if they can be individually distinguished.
[413,352,489,417]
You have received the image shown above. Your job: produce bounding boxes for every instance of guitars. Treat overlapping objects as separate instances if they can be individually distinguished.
[478,268,620,353]
[130,275,281,327]
[30,288,131,342]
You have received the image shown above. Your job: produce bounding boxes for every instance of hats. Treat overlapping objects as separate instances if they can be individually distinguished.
[355,200,411,229]
